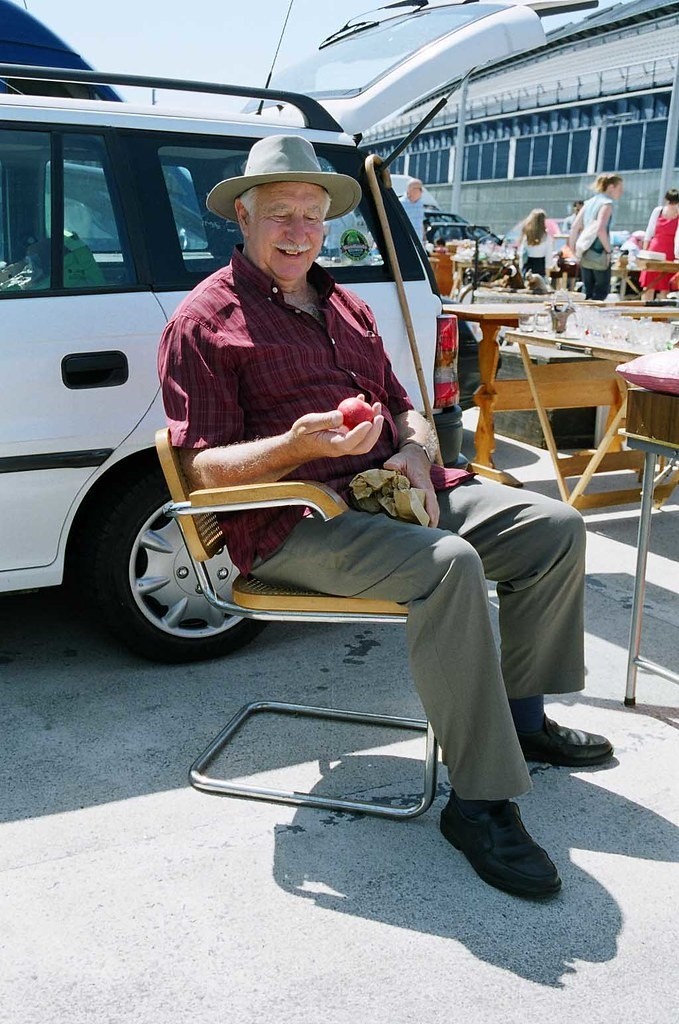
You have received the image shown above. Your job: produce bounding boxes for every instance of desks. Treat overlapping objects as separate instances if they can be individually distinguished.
[508,328,678,510]
[442,305,678,488]
[637,258,679,299]
[617,386,678,707]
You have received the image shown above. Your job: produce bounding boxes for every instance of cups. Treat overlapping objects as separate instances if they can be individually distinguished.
[517,304,679,350]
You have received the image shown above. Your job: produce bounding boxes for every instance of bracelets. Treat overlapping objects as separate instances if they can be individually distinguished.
[400,439,431,463]
[606,250,613,254]
[422,241,428,244]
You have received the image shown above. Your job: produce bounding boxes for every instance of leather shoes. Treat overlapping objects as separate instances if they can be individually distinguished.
[439,788,562,899]
[517,712,614,767]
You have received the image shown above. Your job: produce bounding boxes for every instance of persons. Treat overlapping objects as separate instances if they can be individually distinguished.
[568,173,623,301]
[639,188,679,301]
[620,231,644,292]
[562,201,584,288]
[399,180,428,254]
[159,136,614,897]
[520,209,551,276]
[424,220,447,253]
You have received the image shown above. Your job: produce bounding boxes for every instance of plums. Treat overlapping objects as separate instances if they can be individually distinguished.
[336,397,374,430]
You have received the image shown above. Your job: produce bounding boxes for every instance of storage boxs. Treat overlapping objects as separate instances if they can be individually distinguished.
[0,231,108,291]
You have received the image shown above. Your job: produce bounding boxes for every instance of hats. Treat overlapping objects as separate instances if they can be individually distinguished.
[206,135,363,222]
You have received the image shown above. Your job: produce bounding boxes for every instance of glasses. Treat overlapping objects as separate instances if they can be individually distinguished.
[414,187,422,191]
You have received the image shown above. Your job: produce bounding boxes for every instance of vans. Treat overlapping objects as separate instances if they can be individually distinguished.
[1,0,602,666]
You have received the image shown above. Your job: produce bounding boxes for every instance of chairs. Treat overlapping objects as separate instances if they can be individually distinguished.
[155,428,437,818]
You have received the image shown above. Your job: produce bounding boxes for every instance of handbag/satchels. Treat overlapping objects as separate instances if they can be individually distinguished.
[580,248,610,270]
